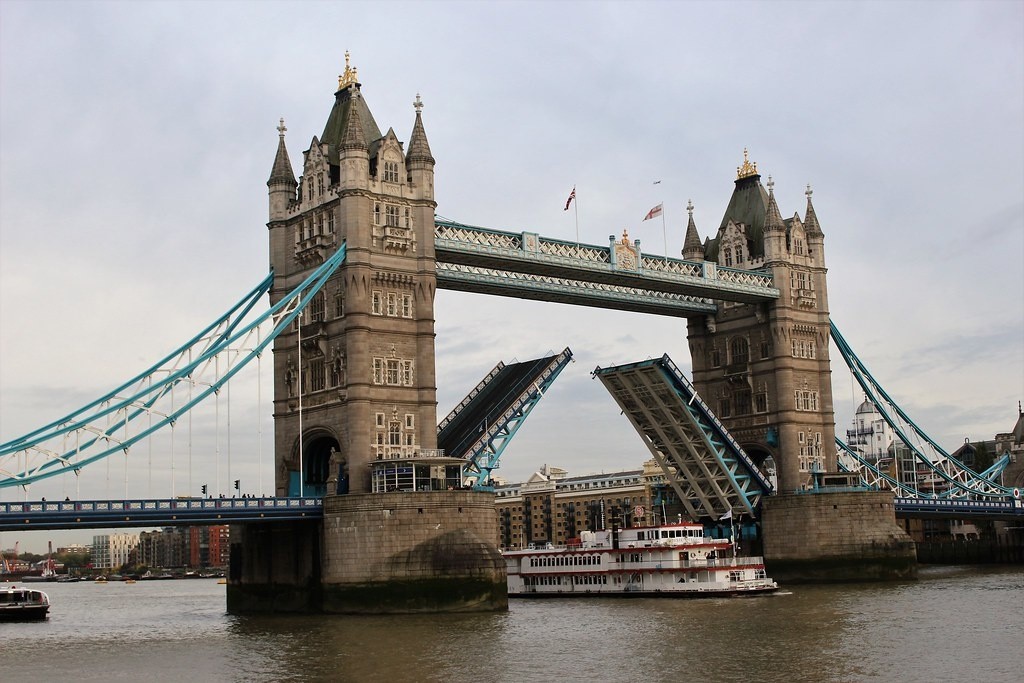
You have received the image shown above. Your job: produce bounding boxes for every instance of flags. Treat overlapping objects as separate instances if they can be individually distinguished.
[564,189,575,211]
[642,204,663,222]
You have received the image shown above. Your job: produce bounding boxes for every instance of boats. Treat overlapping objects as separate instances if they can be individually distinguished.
[0,586,51,620]
[500,503,782,597]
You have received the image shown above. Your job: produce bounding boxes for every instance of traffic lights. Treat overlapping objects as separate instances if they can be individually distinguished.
[201,485,205,494]
[235,480,238,489]
